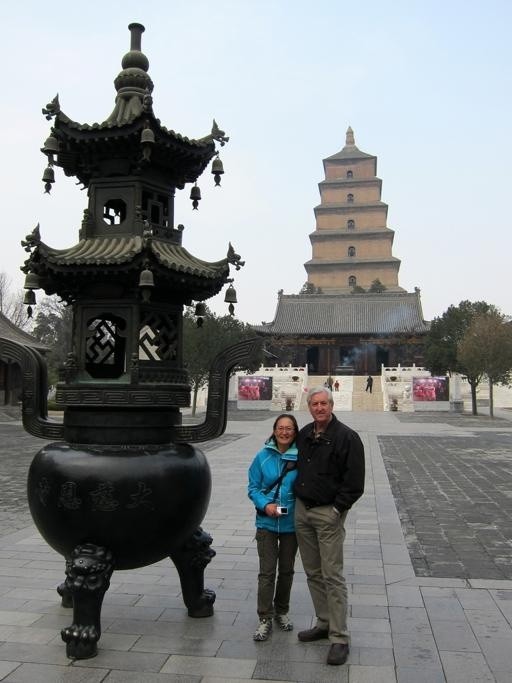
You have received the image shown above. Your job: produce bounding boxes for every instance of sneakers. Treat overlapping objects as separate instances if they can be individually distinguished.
[297,625,330,643]
[252,618,274,642]
[276,612,296,632]
[326,640,350,667]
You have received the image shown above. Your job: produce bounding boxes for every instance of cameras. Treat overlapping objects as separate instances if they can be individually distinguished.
[277,507,289,515]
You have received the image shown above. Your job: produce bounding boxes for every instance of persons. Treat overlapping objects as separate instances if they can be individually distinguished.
[247,414,301,642]
[293,387,366,666]
[326,374,334,392]
[365,374,373,393]
[335,381,340,391]
